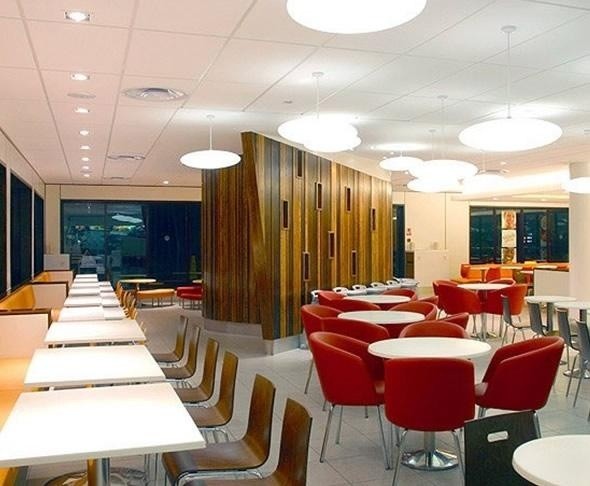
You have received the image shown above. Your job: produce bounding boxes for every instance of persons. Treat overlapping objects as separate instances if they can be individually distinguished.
[504,247,515,263]
[504,211,515,228]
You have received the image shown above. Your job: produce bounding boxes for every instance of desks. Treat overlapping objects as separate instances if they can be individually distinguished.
[24,344,168,391]
[523,295,578,334]
[0,381,206,486]
[512,433,590,486]
[0,272,206,486]
[554,301,590,322]
[43,276,147,348]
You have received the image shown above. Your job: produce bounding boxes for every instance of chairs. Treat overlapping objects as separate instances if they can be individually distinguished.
[152,315,190,368]
[557,308,580,376]
[152,349,239,485]
[300,262,569,486]
[525,299,548,338]
[500,293,533,346]
[162,373,278,484]
[463,410,541,485]
[183,397,317,485]
[575,318,589,422]
[160,323,201,389]
[172,337,221,407]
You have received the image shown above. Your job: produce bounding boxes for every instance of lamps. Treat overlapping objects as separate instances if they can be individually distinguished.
[406,160,519,196]
[458,22,564,151]
[285,0,431,34]
[178,114,243,172]
[378,147,423,171]
[278,71,363,153]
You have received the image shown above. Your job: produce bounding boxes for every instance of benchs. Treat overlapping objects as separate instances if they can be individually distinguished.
[1,271,75,485]
